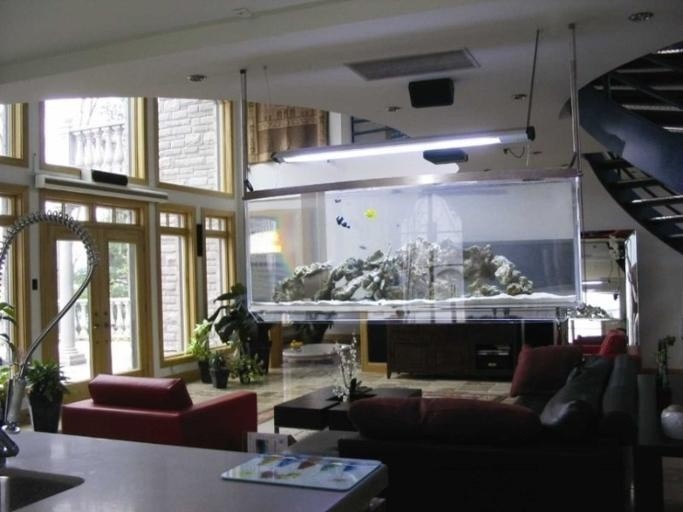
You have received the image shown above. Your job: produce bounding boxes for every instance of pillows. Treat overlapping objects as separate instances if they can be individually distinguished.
[508,342,613,426]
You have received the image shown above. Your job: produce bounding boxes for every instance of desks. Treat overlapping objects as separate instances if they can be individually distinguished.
[635,368,683,511]
[0,421,392,512]
[281,342,340,365]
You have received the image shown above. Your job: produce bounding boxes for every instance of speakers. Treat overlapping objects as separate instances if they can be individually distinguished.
[408,77,455,108]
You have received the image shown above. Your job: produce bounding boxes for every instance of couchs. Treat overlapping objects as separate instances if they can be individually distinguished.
[59,371,258,453]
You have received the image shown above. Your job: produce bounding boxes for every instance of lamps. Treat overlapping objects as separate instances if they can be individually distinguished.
[269,27,544,167]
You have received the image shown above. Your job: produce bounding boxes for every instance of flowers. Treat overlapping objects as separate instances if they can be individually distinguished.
[334,334,356,388]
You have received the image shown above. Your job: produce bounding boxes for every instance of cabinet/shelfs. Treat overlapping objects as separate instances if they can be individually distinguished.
[384,318,571,384]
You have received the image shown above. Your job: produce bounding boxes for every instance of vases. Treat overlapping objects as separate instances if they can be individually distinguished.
[338,370,353,401]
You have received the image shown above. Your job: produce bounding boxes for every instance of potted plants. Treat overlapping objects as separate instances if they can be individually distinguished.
[0,359,71,434]
[183,281,275,389]
